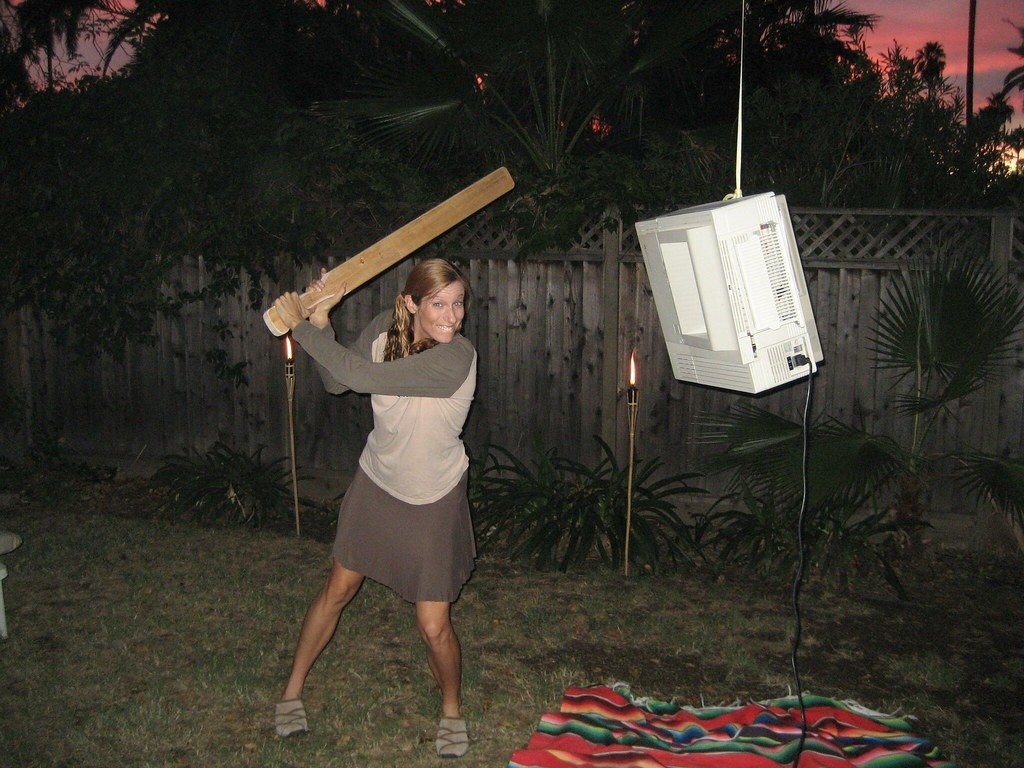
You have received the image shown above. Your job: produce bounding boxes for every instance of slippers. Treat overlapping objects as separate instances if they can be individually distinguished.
[436,718,469,757]
[275,699,308,737]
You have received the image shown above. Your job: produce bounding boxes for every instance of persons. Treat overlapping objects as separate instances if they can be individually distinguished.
[271,259,478,757]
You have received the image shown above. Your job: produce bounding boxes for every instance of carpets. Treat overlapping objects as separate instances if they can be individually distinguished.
[508,682,956,768]
